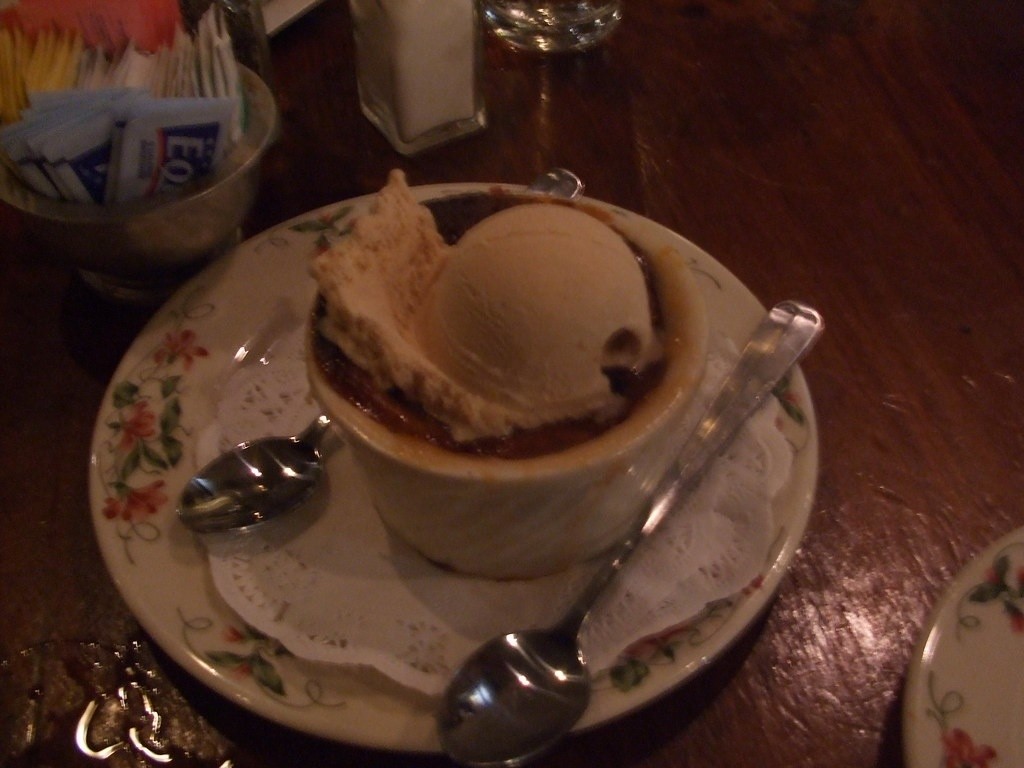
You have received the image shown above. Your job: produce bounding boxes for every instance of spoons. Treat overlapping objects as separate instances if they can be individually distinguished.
[176,167,585,535]
[434,300,827,767]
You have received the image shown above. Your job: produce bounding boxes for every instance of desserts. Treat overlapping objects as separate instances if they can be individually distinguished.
[312,168,668,459]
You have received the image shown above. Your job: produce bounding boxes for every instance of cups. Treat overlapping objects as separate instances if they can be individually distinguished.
[305,192,709,580]
[179,0,272,95]
[481,0,622,54]
[350,1,487,156]
[0,63,276,304]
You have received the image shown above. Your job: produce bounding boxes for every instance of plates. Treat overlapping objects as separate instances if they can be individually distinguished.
[89,182,819,754]
[902,526,1024,767]
